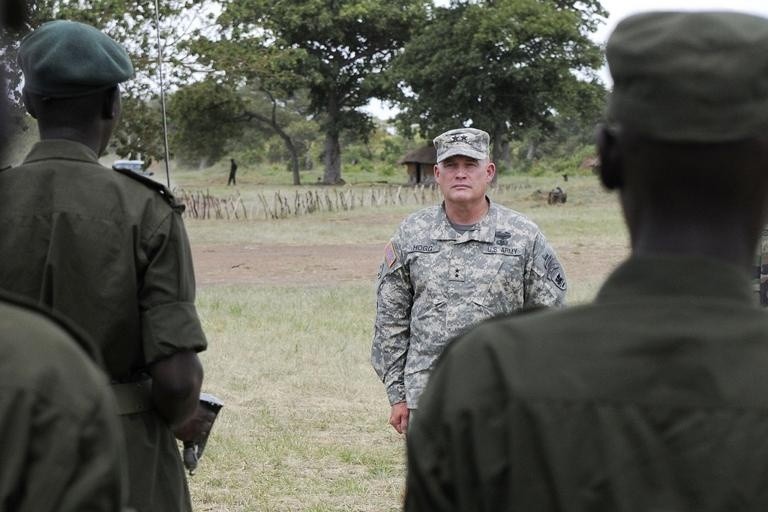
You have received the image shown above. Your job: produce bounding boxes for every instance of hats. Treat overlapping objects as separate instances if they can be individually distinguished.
[606,12,768,143]
[432,128,489,163]
[17,20,133,86]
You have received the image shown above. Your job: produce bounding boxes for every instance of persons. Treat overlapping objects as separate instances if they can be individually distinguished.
[749,227,768,305]
[227,160,237,184]
[0,59,131,512]
[401,9,768,512]
[369,128,567,435]
[0,20,215,512]
